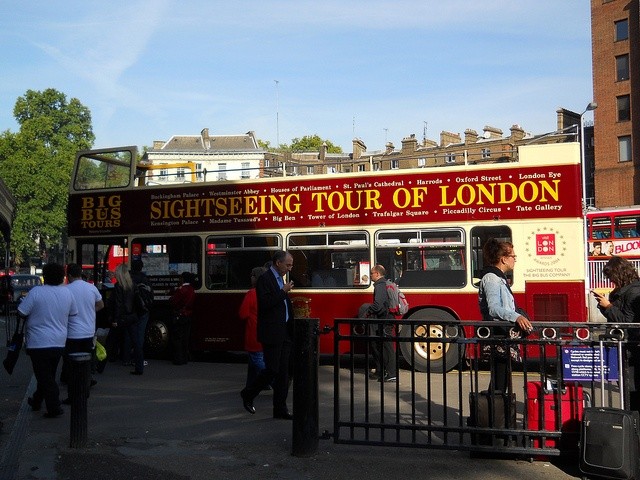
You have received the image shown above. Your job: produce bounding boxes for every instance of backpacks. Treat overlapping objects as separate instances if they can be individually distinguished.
[384,278,408,316]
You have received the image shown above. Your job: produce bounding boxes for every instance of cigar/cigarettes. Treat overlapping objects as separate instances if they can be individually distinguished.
[527,327,531,330]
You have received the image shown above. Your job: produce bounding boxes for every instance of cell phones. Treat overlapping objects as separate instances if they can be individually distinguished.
[590,291,601,297]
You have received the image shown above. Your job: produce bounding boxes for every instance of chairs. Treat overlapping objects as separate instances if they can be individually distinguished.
[630,229,640,237]
[610,228,625,238]
[595,230,607,239]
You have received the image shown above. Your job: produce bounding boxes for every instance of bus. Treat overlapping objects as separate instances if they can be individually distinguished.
[585,205,640,290]
[97,231,485,285]
[63,140,593,378]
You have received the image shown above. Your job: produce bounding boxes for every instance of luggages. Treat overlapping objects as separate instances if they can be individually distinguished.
[577,334,639,478]
[524,378,583,465]
[468,390,520,461]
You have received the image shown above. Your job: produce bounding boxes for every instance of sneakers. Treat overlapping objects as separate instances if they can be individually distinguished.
[27,397,41,411]
[43,399,62,419]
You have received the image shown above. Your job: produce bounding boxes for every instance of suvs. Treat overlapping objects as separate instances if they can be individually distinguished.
[63,263,94,287]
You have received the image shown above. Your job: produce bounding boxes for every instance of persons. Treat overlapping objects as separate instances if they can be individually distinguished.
[16,264,75,418]
[173,271,195,364]
[479,240,533,395]
[112,259,154,374]
[240,252,294,419]
[591,242,605,257]
[367,264,398,381]
[239,268,273,391]
[607,240,614,253]
[60,262,104,405]
[593,257,639,411]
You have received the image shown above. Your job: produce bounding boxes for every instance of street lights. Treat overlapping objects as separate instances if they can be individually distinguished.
[578,102,600,320]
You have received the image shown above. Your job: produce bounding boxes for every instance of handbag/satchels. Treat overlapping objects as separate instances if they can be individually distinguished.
[3,315,29,375]
[498,308,536,341]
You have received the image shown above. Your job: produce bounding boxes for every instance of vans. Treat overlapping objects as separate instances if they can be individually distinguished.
[1,274,43,315]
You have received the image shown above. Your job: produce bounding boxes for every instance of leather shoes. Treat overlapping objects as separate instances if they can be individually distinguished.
[272,410,295,420]
[384,377,397,383]
[240,390,256,415]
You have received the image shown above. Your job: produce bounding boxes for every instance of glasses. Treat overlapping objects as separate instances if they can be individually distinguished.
[508,253,518,262]
